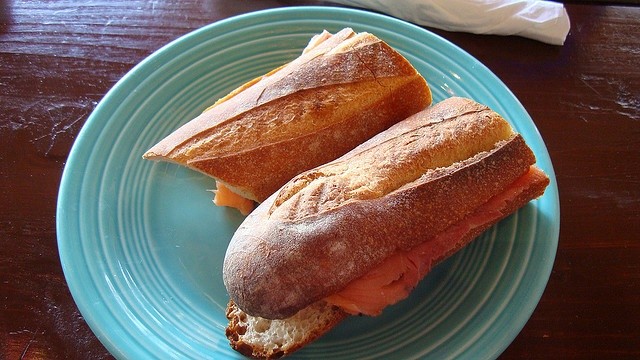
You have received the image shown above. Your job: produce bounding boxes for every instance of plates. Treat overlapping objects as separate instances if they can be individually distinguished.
[56,6,562,359]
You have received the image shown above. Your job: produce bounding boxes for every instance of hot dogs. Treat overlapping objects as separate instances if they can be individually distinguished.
[224,97,551,360]
[142,27,430,217]
